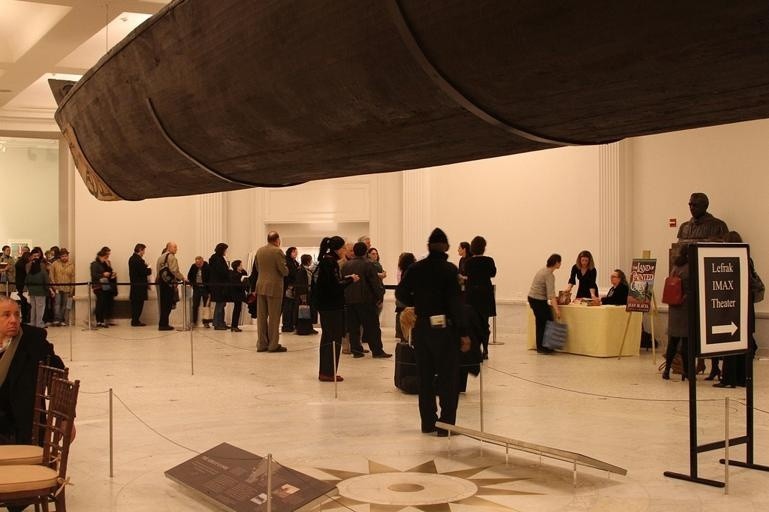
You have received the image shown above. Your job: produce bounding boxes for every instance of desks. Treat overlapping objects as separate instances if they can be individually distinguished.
[525,302,644,358]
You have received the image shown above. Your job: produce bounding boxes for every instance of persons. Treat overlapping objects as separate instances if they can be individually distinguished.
[696,236,723,382]
[676,192,729,238]
[712,230,758,389]
[0,295,76,511]
[663,243,691,380]
[565,250,598,299]
[527,253,562,353]
[592,269,629,305]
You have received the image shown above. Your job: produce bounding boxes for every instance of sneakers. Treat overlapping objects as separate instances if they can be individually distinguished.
[319,374,343,381]
[373,350,393,358]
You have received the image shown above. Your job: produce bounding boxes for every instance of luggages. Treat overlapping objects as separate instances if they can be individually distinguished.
[395,325,420,393]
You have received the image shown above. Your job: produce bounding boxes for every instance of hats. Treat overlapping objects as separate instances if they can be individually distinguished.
[429,228,447,242]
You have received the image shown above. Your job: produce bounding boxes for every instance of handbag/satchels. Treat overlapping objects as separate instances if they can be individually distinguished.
[201,306,215,320]
[543,321,567,350]
[160,263,177,287]
[751,272,765,303]
[298,304,311,320]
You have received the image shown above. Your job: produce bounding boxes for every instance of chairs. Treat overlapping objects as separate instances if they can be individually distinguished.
[1,360,70,512]
[1,372,81,512]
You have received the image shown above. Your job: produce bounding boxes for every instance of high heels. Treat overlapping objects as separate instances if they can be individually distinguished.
[231,327,241,331]
[695,365,736,389]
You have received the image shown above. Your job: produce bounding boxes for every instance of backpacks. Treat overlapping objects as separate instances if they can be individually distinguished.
[662,275,687,304]
